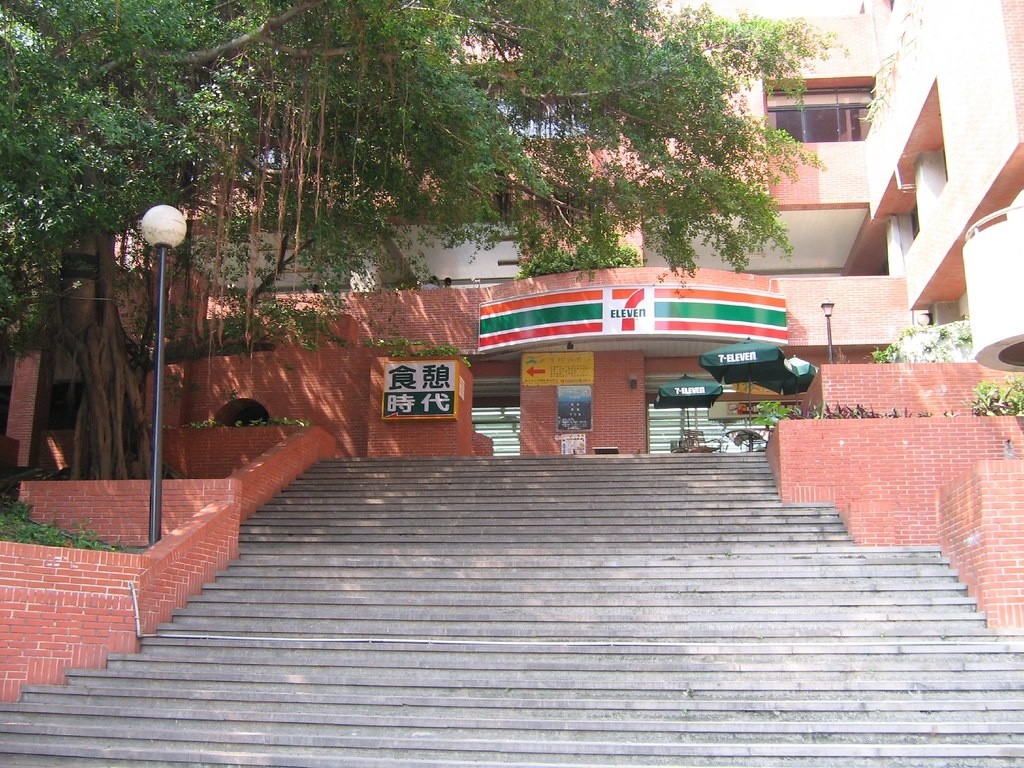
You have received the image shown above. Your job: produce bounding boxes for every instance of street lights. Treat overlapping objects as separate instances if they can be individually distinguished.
[139,206,189,547]
[819,299,836,364]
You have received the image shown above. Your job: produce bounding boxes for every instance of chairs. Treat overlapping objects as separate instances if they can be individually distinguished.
[670,429,721,453]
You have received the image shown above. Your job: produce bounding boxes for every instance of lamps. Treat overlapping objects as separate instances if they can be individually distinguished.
[916,313,934,325]
[629,372,639,389]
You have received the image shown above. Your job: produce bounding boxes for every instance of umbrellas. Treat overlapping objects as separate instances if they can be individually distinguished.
[698,336,820,426]
[654,374,724,445]
